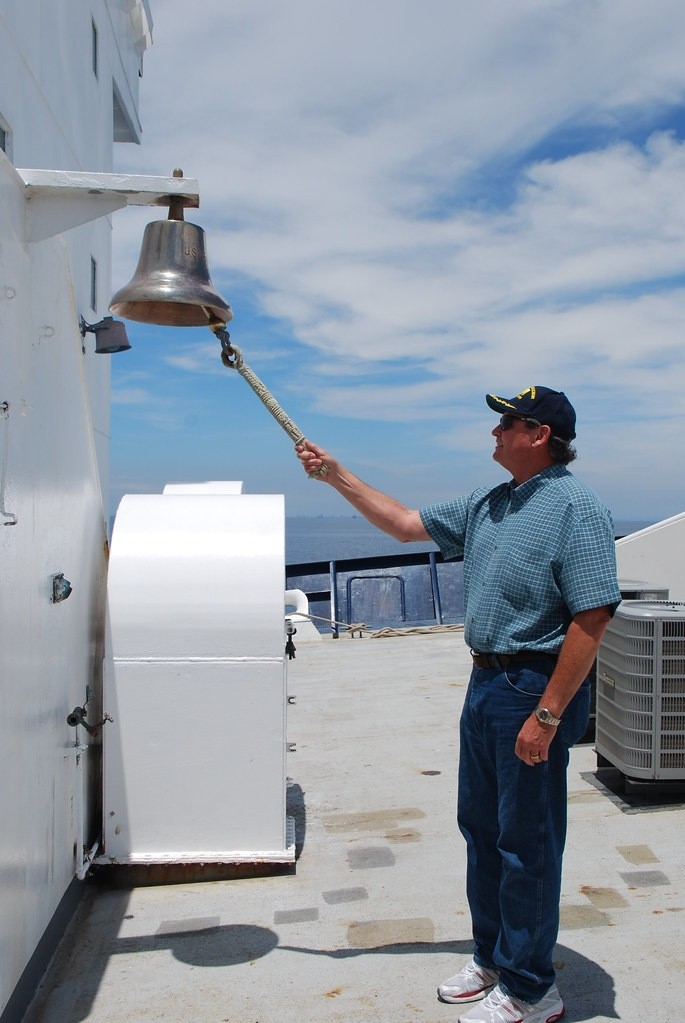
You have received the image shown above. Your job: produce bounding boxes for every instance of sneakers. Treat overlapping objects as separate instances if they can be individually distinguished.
[437,957,502,1003]
[457,981,566,1023]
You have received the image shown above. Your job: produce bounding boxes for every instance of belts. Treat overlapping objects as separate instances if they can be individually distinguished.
[470,648,552,669]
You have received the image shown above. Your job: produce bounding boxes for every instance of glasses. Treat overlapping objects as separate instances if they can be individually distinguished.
[499,415,541,432]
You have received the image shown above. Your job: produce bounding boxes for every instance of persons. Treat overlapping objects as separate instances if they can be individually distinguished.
[294,386,620,1023]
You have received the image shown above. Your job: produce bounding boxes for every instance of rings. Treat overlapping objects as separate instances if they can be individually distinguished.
[530,755,540,759]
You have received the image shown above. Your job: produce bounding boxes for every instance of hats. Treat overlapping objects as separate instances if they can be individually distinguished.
[485,385,578,446]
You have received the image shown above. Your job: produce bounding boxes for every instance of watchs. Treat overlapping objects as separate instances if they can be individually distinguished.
[534,705,561,726]
